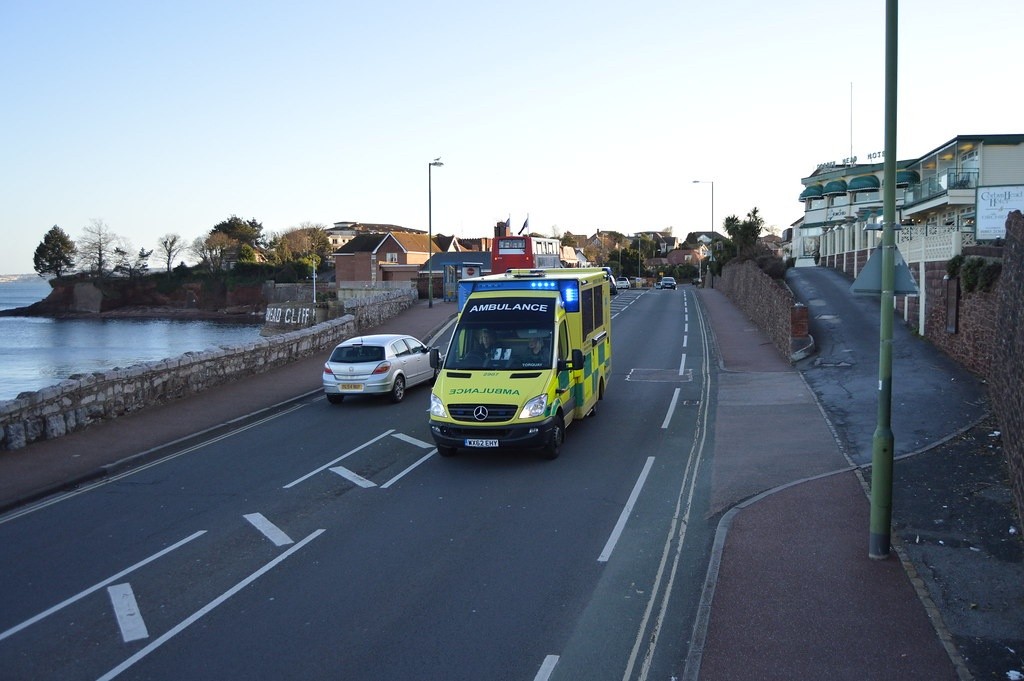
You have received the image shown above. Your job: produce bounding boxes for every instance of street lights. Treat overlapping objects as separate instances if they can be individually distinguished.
[692,180,715,290]
[428,161,444,309]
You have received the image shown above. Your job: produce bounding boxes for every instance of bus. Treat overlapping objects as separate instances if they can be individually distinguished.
[492,235,560,274]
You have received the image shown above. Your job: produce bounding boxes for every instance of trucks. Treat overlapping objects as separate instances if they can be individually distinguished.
[425,265,613,462]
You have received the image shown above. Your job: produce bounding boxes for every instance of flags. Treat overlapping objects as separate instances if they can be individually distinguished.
[505,218,510,227]
[518,218,527,235]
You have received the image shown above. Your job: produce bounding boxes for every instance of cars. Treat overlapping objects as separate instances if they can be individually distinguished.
[608,275,618,295]
[615,278,631,289]
[660,276,678,290]
[321,332,445,404]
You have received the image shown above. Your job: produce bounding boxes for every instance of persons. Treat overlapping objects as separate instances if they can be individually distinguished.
[520,332,550,370]
[463,327,513,367]
